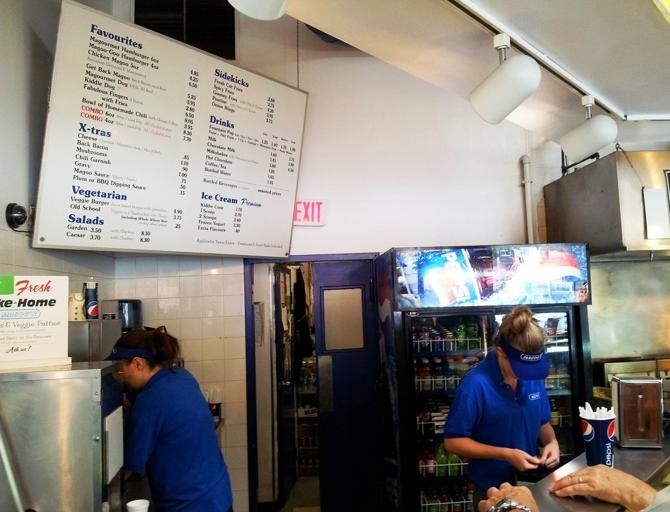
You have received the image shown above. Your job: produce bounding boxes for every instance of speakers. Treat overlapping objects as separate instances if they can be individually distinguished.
[134,0,236,61]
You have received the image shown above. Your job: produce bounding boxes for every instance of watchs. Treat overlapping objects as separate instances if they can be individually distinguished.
[487,497,531,512]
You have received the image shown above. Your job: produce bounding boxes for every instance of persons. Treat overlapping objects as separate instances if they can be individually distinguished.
[477,462,670,512]
[102,328,234,512]
[442,307,560,512]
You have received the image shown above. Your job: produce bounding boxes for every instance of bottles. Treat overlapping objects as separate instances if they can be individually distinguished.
[455,315,479,350]
[83,282,99,320]
[125,497,150,512]
[546,356,579,427]
[411,354,480,394]
[420,436,469,477]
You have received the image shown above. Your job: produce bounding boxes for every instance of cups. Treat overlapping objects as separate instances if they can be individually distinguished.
[579,413,617,468]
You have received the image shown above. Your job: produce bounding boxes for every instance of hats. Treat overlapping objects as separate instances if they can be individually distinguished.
[496,336,550,381]
[102,344,150,360]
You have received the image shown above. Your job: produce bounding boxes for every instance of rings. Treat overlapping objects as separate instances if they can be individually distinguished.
[579,477,583,483]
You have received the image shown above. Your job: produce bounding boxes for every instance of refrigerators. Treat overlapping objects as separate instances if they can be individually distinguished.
[373,240,597,511]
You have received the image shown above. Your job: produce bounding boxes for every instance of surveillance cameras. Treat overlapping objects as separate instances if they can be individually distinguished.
[5,203,27,229]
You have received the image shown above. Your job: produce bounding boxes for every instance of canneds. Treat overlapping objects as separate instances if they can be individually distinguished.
[412,324,455,353]
